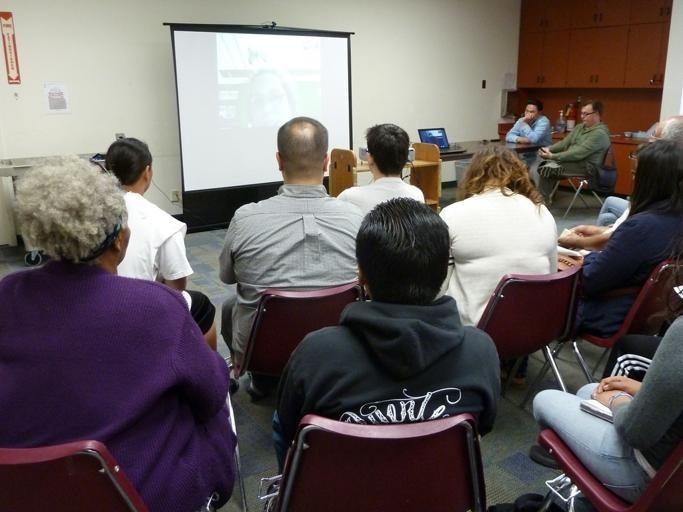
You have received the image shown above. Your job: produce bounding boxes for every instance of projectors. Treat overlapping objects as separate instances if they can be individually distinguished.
[358,146,415,162]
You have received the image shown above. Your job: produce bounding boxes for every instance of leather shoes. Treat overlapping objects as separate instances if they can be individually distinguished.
[529,443,560,467]
[247,371,268,401]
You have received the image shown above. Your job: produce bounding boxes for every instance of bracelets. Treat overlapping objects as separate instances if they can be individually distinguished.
[609,391,634,410]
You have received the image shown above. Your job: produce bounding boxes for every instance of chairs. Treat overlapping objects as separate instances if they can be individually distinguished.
[476,264,595,399]
[517,259,683,410]
[257,414,486,512]
[224,281,364,404]
[1,440,219,512]
[513,426,682,512]
[547,145,617,220]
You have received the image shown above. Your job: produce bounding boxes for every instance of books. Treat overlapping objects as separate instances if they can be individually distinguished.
[581,400,614,423]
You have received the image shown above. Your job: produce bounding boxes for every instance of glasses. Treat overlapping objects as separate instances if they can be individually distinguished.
[579,112,598,117]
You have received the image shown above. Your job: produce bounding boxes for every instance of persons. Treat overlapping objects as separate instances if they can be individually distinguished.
[338,125,425,218]
[505,100,552,190]
[531,335,663,469]
[220,117,364,403]
[502,141,683,386]
[439,143,558,329]
[0,151,237,511]
[597,144,650,226]
[533,240,683,505]
[105,137,216,353]
[559,116,682,252]
[537,100,611,211]
[265,197,501,512]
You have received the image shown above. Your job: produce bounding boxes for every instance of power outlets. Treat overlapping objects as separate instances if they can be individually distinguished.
[169,190,180,203]
[115,133,125,141]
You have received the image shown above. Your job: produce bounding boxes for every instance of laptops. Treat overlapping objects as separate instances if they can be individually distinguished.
[417,127,466,153]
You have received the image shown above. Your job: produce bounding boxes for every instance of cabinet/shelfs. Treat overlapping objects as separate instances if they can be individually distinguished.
[499,123,659,196]
[623,22,670,89]
[518,30,568,88]
[570,0,631,29]
[521,0,571,32]
[629,0,672,24]
[329,143,442,215]
[568,26,631,88]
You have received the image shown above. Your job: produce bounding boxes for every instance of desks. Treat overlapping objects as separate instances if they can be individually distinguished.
[359,138,545,164]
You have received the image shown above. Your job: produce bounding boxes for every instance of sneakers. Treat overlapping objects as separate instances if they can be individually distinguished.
[227,378,237,394]
[539,163,564,177]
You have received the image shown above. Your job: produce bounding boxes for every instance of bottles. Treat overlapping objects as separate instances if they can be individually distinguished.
[565,104,577,133]
[557,110,566,132]
